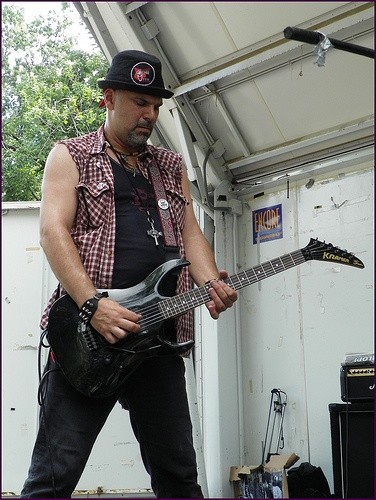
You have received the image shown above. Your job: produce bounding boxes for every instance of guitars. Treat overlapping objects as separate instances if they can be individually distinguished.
[48,236,366,399]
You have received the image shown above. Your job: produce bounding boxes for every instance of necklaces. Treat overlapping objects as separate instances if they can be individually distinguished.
[105,141,148,176]
[102,131,163,246]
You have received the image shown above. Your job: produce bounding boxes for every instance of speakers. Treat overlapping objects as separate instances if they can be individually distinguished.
[329,402,375,498]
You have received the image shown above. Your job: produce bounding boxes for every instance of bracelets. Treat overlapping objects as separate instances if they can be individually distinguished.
[79,291,109,324]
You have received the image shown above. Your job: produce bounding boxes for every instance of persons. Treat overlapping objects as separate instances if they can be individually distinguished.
[19,51,239,500]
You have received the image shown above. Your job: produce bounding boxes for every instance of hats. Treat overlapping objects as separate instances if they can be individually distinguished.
[97,50,174,99]
